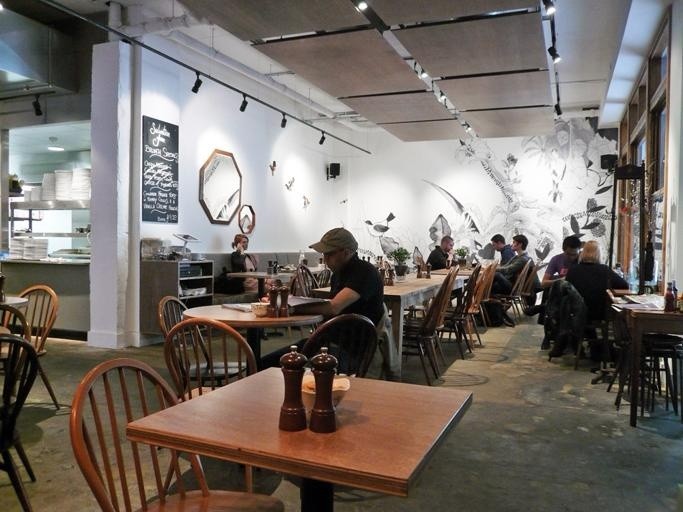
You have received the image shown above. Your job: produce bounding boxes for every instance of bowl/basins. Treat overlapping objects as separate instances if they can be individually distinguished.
[300,375,350,410]
[422,271,428,278]
[76,227,88,233]
[251,302,270,317]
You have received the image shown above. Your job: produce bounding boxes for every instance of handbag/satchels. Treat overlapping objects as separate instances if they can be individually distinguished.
[214,266,244,295]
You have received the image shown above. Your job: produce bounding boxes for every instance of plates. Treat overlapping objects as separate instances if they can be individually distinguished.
[23,190,31,202]
[33,239,49,261]
[72,168,91,201]
[9,237,25,259]
[30,186,42,202]
[23,238,35,261]
[55,169,71,200]
[41,172,55,200]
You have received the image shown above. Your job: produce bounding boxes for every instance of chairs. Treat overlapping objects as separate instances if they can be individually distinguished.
[157,294,248,450]
[538,279,682,417]
[163,317,259,495]
[379,258,542,386]
[302,312,378,378]
[0,284,60,512]
[69,357,285,512]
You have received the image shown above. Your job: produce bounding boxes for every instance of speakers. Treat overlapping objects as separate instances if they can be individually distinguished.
[600,153,617,172]
[329,162,340,180]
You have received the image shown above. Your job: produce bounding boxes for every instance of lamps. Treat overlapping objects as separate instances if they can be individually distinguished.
[31,94,45,118]
[190,72,325,144]
[326,163,340,180]
[356,0,472,133]
[543,0,564,116]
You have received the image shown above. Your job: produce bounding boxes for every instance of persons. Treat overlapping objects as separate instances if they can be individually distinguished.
[490,234,515,266]
[232,234,274,294]
[565,240,629,358]
[426,235,459,270]
[485,234,528,326]
[540,236,582,357]
[259,226,383,374]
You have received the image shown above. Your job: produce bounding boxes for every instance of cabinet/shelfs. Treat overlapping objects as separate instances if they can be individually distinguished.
[140,258,215,336]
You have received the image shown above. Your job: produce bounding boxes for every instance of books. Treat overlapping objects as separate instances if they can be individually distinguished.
[277,295,333,308]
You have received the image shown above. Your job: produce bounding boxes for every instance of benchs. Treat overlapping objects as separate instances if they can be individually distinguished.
[203,251,321,305]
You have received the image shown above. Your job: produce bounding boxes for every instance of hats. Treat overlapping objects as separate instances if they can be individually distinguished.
[309,227,358,254]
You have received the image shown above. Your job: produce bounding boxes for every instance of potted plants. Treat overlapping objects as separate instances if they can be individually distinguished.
[455,248,467,266]
[391,247,411,277]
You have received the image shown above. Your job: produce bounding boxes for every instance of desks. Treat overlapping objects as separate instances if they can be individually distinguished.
[181,301,324,378]
[227,266,331,341]
[124,365,473,512]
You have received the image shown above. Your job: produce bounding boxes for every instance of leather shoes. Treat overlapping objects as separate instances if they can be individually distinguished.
[541,337,550,350]
[524,305,539,316]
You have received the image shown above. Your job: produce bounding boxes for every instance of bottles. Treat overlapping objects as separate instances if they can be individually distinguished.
[417,264,422,278]
[273,261,279,275]
[380,269,387,286]
[387,269,394,286]
[663,282,674,312]
[362,256,365,261]
[613,263,625,279]
[278,345,307,431]
[377,256,380,264]
[320,252,325,264]
[380,256,383,265]
[266,287,279,318]
[279,287,290,318]
[303,259,308,265]
[307,345,337,433]
[299,250,305,264]
[679,293,683,313]
[627,260,638,292]
[446,260,450,269]
[672,280,678,311]
[0,275,6,303]
[426,263,432,279]
[269,261,273,267]
[368,257,370,263]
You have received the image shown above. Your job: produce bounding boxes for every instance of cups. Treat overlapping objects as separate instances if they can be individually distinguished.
[267,267,273,275]
[318,264,325,271]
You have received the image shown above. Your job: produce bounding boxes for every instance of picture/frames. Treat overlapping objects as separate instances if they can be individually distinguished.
[200,148,242,225]
[238,204,256,234]
